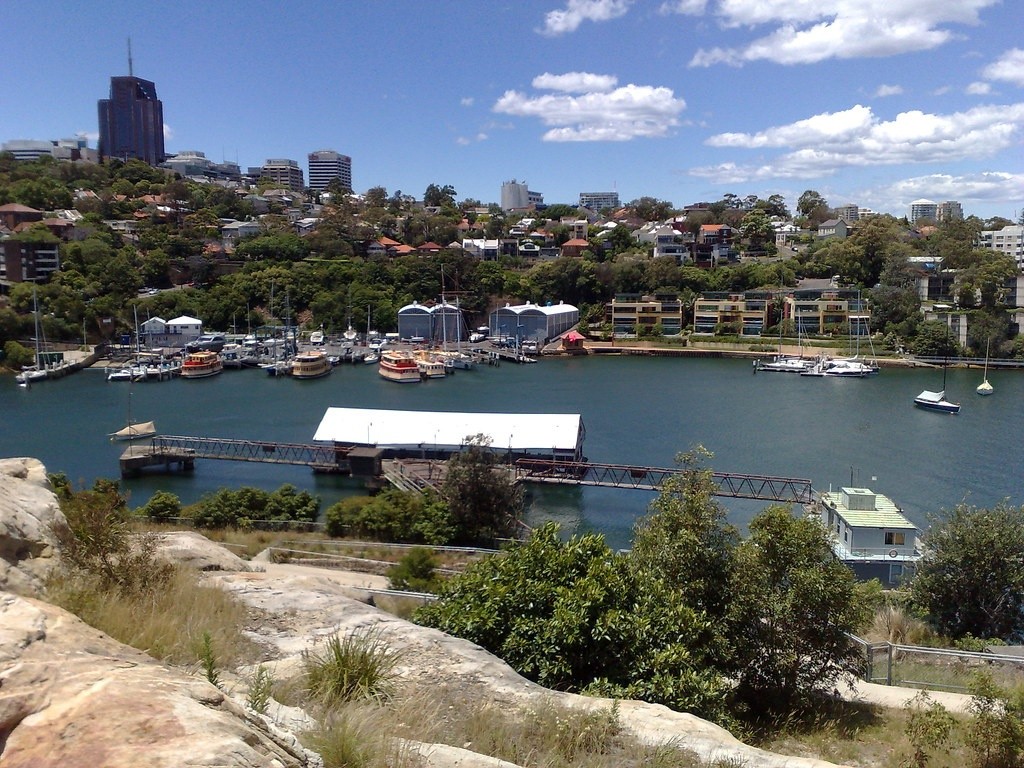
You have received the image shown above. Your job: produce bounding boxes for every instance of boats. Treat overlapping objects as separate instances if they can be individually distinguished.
[102,264,475,383]
[913,390,961,413]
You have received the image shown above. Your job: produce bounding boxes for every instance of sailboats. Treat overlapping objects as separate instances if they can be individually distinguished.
[976,337,994,396]
[756,289,881,378]
[15,282,48,381]
